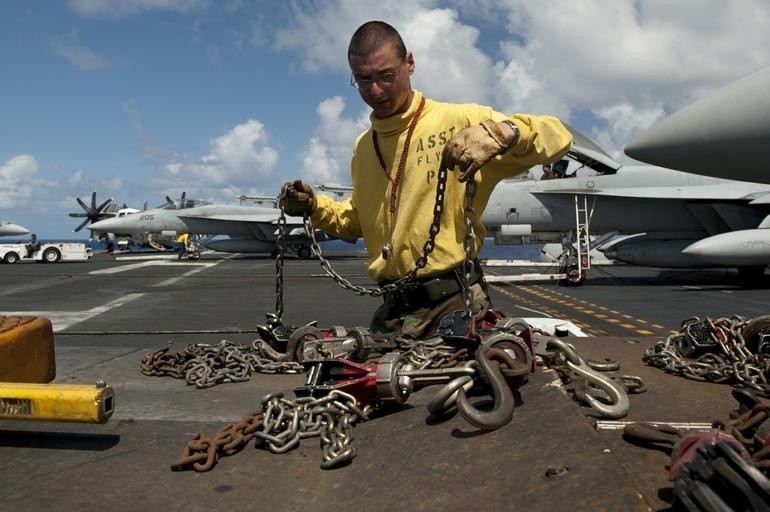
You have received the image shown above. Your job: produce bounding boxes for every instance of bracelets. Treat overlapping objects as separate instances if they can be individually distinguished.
[502,120,520,149]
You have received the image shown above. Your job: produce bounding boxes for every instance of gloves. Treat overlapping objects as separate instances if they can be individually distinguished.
[441,118,509,182]
[280,179,316,217]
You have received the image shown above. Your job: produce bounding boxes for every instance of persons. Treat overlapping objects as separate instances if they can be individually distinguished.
[280,20,581,323]
[176,233,191,259]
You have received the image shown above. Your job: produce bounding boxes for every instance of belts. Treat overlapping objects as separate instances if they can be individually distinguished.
[378,257,484,312]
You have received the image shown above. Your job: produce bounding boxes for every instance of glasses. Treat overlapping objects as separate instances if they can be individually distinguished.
[351,59,405,90]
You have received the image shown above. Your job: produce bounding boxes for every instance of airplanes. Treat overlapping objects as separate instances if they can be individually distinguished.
[0,218,29,235]
[483,68,770,273]
[70,192,357,260]
